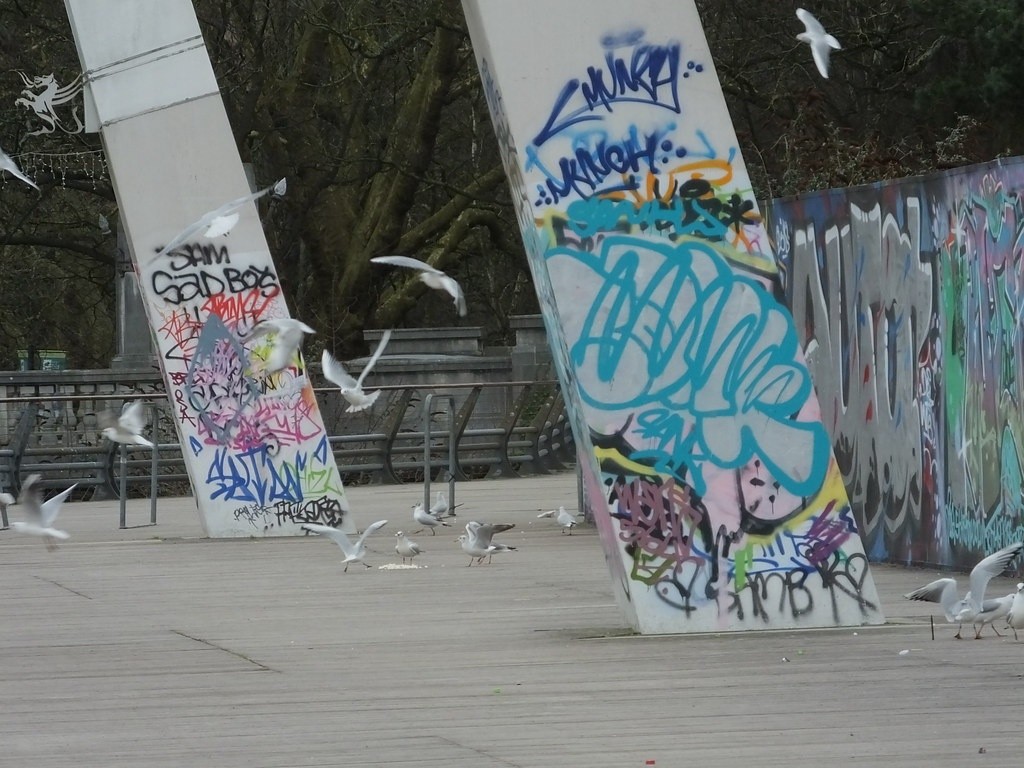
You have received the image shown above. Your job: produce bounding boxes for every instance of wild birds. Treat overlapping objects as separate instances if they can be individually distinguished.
[0,150,581,573]
[905,543,1024,639]
[796,8,842,79]
